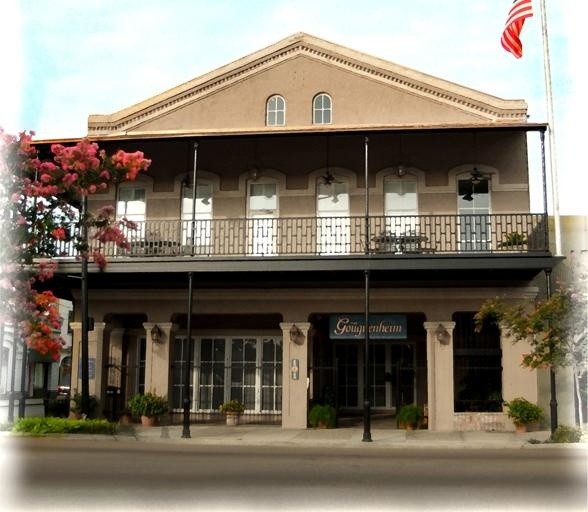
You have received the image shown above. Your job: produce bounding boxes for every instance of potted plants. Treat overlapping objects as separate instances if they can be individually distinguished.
[68,393,83,420]
[128,392,169,425]
[308,404,337,429]
[395,404,423,430]
[217,400,246,425]
[503,397,545,432]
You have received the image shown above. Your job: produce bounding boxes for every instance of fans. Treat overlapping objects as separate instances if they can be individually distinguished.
[177,141,208,188]
[456,131,495,182]
[317,134,343,186]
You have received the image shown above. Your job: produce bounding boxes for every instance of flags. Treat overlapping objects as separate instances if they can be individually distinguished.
[500,0,534,59]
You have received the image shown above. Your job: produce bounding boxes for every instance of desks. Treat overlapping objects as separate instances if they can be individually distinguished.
[371,236,429,252]
[120,241,181,255]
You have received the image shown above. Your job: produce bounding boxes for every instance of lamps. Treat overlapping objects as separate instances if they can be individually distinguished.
[435,324,448,342]
[290,324,299,343]
[151,324,161,343]
[392,134,408,178]
